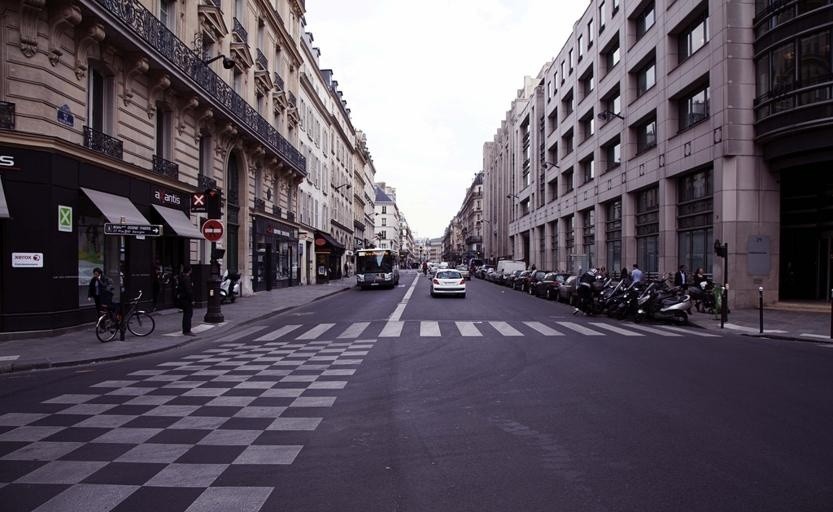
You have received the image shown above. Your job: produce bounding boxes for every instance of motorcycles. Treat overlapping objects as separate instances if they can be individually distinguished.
[217,271,241,305]
[593,276,702,325]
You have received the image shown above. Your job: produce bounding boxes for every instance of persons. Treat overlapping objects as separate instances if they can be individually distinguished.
[572,268,599,316]
[631,265,644,284]
[694,267,713,312]
[423,261,427,277]
[177,265,196,336]
[600,266,607,277]
[675,265,691,314]
[87,268,113,333]
[620,269,632,286]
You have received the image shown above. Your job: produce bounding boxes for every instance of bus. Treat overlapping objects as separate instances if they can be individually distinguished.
[355,248,399,291]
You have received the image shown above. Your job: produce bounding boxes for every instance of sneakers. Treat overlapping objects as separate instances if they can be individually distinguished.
[183,330,197,337]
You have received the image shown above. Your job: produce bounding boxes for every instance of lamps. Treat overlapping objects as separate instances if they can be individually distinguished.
[205,55,235,69]
[542,161,559,169]
[506,193,519,199]
[368,212,378,216]
[335,183,352,192]
[598,110,624,121]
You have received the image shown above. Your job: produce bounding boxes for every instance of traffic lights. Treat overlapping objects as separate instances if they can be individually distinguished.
[213,250,225,259]
[206,189,221,219]
[192,194,205,207]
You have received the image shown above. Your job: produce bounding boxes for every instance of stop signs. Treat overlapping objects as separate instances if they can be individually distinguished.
[202,219,224,241]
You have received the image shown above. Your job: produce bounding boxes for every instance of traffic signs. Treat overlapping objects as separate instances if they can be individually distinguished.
[104,223,162,238]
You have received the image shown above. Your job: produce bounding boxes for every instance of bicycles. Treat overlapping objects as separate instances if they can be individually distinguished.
[94,290,155,342]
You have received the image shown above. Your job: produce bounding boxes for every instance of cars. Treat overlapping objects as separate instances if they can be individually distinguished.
[454,265,471,280]
[469,258,603,307]
[426,260,466,298]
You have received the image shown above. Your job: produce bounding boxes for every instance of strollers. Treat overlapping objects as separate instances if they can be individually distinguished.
[701,282,729,315]
